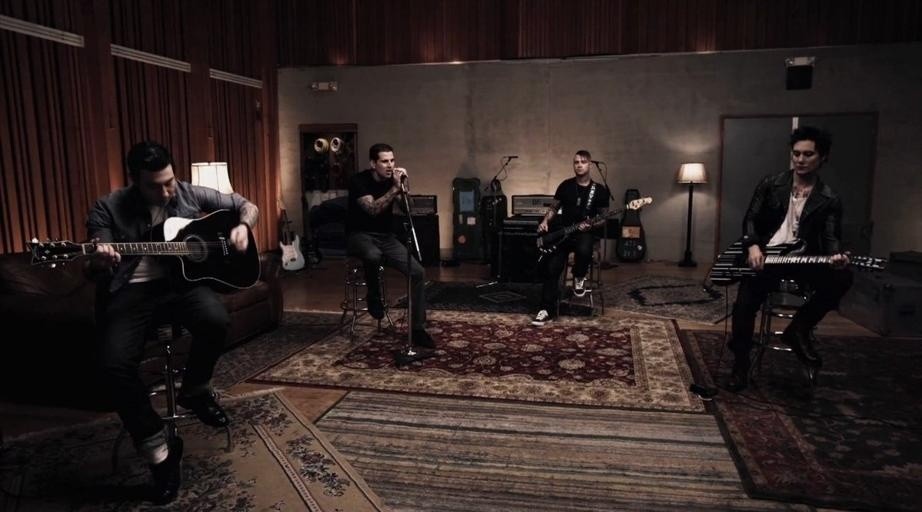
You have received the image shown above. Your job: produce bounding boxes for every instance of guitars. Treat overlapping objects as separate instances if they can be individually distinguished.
[25,211,261,294]
[279,208,307,272]
[709,237,887,285]
[299,196,322,264]
[537,197,652,254]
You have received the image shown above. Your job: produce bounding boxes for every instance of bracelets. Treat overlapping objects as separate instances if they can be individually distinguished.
[235,221,251,232]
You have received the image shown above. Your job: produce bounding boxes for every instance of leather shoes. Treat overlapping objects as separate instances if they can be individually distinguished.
[728,360,751,390]
[412,326,437,349]
[780,327,824,364]
[156,436,184,504]
[177,387,228,426]
[366,279,384,320]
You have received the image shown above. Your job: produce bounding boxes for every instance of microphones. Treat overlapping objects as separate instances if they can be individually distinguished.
[505,156,518,158]
[591,161,599,164]
[395,170,407,183]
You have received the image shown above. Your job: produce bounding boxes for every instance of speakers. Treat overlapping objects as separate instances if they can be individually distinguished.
[491,232,568,284]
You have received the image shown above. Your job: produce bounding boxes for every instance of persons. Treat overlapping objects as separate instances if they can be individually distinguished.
[343,143,437,350]
[724,127,852,395]
[84,139,260,505]
[530,149,609,328]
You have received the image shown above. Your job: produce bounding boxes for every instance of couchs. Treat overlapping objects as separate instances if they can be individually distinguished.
[1,252,284,410]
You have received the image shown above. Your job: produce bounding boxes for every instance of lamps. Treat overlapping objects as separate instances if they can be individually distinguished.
[783,56,818,66]
[191,160,234,194]
[310,81,338,92]
[676,162,707,267]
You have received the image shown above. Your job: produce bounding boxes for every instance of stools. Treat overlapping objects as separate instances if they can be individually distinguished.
[555,239,604,318]
[338,256,396,345]
[112,323,232,481]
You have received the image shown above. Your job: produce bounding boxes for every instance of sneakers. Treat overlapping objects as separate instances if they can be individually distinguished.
[575,278,586,298]
[531,310,553,326]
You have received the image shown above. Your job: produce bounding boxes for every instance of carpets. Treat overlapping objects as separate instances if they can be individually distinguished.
[1,388,384,512]
[150,308,362,408]
[246,274,922,512]
